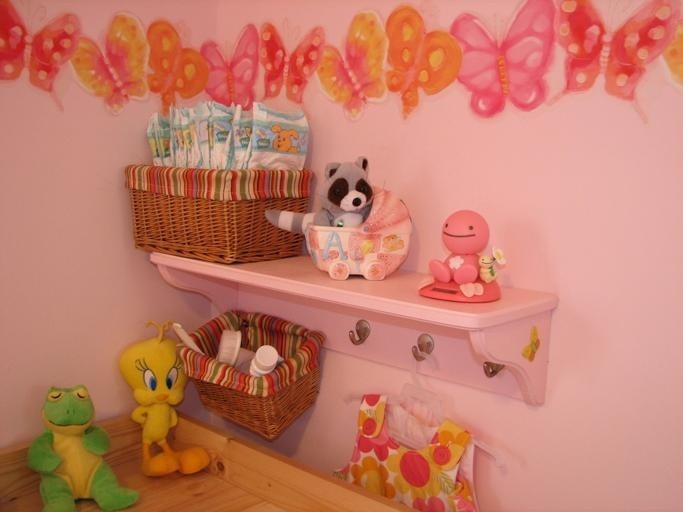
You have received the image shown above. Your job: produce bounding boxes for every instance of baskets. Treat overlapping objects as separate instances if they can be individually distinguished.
[124,165,312,264]
[177,308,325,439]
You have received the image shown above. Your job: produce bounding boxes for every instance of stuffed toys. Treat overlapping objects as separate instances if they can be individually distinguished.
[265,156,375,256]
[120,320,211,477]
[27,385,138,512]
